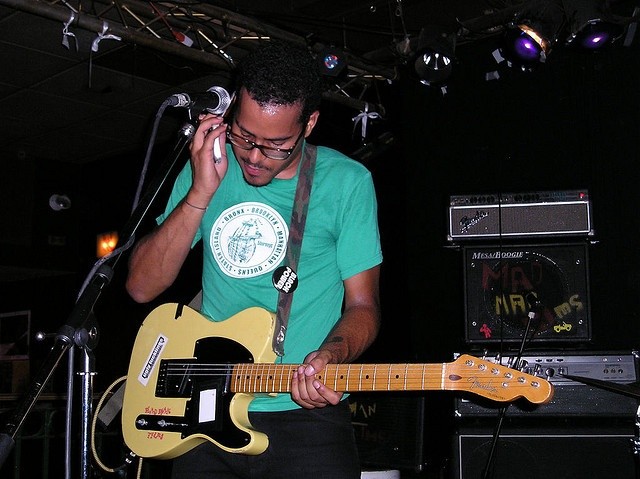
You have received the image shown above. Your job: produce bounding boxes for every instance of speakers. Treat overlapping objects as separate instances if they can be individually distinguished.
[462,241,592,343]
[452,427,640,479]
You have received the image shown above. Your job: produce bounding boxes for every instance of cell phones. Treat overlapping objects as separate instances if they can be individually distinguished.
[212,92,235,163]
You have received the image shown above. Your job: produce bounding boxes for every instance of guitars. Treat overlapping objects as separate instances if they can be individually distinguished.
[122,303,553,461]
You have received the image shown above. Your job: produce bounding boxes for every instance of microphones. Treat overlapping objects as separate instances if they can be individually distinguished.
[173,86,232,115]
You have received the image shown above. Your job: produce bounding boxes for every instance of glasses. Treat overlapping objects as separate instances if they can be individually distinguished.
[225,118,310,160]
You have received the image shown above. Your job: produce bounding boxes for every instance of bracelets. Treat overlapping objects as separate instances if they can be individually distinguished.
[184,196,207,211]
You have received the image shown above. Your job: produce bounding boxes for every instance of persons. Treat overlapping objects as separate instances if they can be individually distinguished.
[125,38,384,479]
